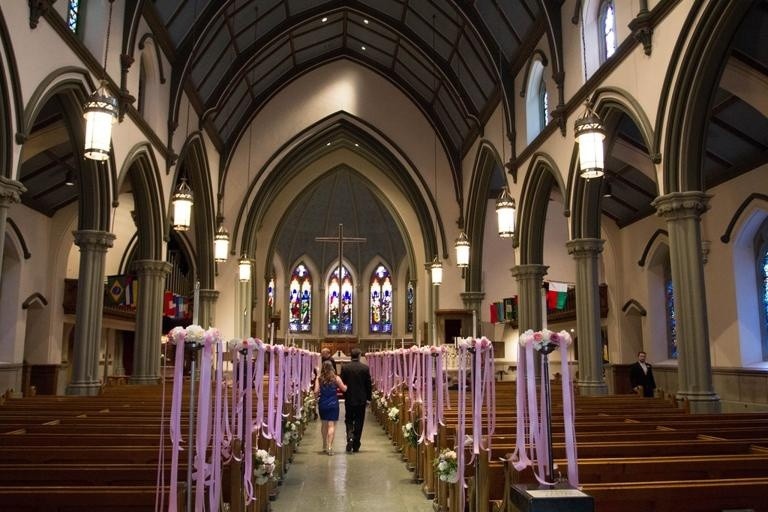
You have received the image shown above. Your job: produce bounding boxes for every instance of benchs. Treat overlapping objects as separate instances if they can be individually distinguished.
[368,376,767,509]
[1,376,316,511]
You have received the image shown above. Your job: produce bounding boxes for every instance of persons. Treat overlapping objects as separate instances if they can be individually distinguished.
[312,360,350,456]
[340,347,374,452]
[318,348,336,373]
[628,350,658,398]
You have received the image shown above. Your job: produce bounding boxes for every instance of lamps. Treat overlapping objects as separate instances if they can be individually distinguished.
[173,1,194,234]
[431,0,472,286]
[574,0,606,180]
[214,0,256,282]
[497,0,517,239]
[84,2,120,162]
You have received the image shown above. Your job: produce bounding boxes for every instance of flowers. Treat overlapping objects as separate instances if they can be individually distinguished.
[168,322,321,359]
[366,328,572,358]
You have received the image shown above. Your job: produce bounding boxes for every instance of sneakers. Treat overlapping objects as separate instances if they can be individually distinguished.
[321,439,359,456]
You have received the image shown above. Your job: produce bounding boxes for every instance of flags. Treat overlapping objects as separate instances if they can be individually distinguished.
[489,282,569,324]
[106,274,191,320]
